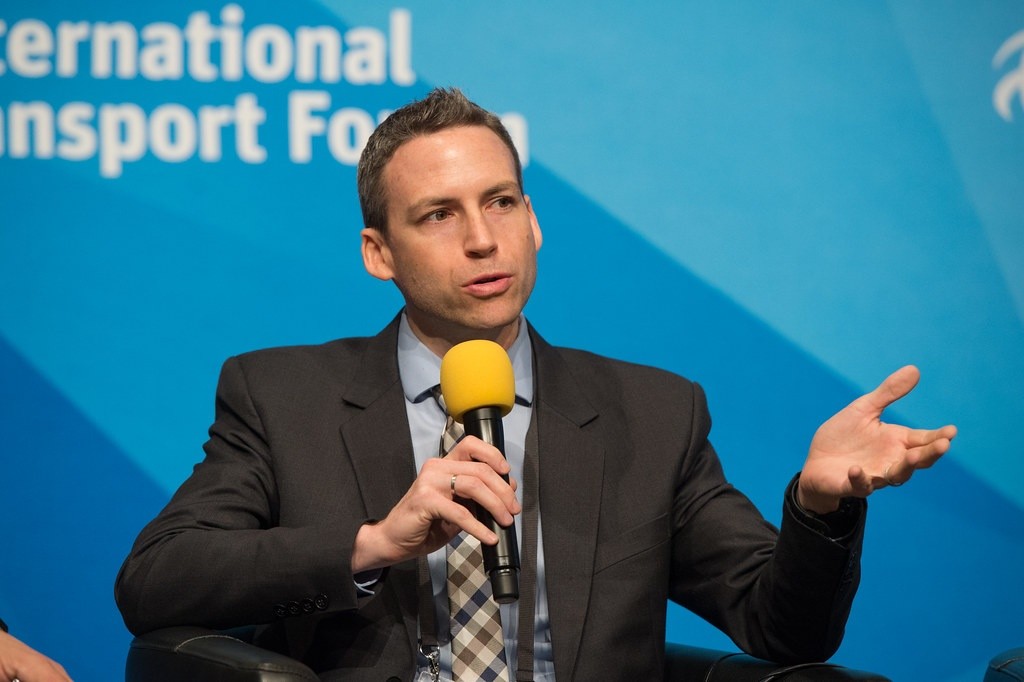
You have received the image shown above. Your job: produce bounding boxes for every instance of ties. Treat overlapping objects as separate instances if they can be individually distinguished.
[431,383,510,682]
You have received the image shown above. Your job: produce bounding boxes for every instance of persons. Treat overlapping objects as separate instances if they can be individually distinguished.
[113,85,958,682]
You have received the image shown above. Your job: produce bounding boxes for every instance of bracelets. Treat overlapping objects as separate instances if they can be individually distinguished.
[790,479,858,536]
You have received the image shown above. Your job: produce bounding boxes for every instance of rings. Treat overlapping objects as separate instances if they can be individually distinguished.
[451,474,458,495]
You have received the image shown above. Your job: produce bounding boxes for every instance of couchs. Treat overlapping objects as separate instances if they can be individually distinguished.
[124,628,894,682]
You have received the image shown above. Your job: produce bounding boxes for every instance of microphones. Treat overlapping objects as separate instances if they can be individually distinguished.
[440,340,523,605]
